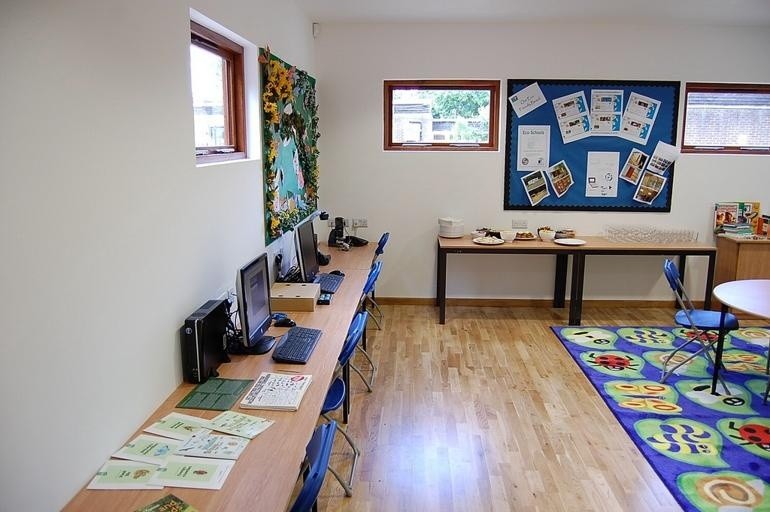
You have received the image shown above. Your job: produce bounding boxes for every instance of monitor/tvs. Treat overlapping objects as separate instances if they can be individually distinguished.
[294,220,318,282]
[231,252,276,355]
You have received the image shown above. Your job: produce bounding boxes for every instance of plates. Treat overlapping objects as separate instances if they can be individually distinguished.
[515,231,538,240]
[473,238,504,244]
[554,239,586,245]
[437,215,465,238]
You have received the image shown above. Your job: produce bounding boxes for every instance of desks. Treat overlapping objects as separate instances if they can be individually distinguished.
[55,236,381,510]
[575,234,721,331]
[711,278,770,405]
[434,229,583,329]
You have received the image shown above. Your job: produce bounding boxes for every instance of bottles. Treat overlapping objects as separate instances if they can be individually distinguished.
[556,229,576,238]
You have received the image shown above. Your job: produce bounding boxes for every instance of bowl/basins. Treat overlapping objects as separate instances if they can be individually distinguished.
[540,230,554,243]
[470,230,485,238]
[501,231,517,242]
[489,232,501,239]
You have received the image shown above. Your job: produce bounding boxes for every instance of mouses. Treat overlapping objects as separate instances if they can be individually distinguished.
[329,270,345,276]
[274,318,296,327]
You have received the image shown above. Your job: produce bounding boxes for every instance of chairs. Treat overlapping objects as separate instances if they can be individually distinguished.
[660,258,742,400]
[290,230,390,511]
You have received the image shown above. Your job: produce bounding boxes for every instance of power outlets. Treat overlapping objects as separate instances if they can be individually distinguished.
[327,218,336,228]
[343,218,349,227]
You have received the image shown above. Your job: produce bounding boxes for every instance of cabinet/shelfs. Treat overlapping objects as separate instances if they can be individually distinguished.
[708,230,770,323]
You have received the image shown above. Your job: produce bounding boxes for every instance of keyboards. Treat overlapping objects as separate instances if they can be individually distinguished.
[272,326,323,363]
[314,273,344,293]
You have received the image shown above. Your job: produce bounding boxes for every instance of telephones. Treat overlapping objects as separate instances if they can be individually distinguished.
[344,236,369,247]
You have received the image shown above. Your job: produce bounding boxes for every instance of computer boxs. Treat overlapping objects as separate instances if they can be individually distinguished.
[182,300,230,385]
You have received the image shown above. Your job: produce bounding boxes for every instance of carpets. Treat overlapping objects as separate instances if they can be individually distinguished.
[545,320,770,512]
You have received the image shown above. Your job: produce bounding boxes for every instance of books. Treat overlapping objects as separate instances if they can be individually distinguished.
[714,201,760,237]
[240,371,312,411]
